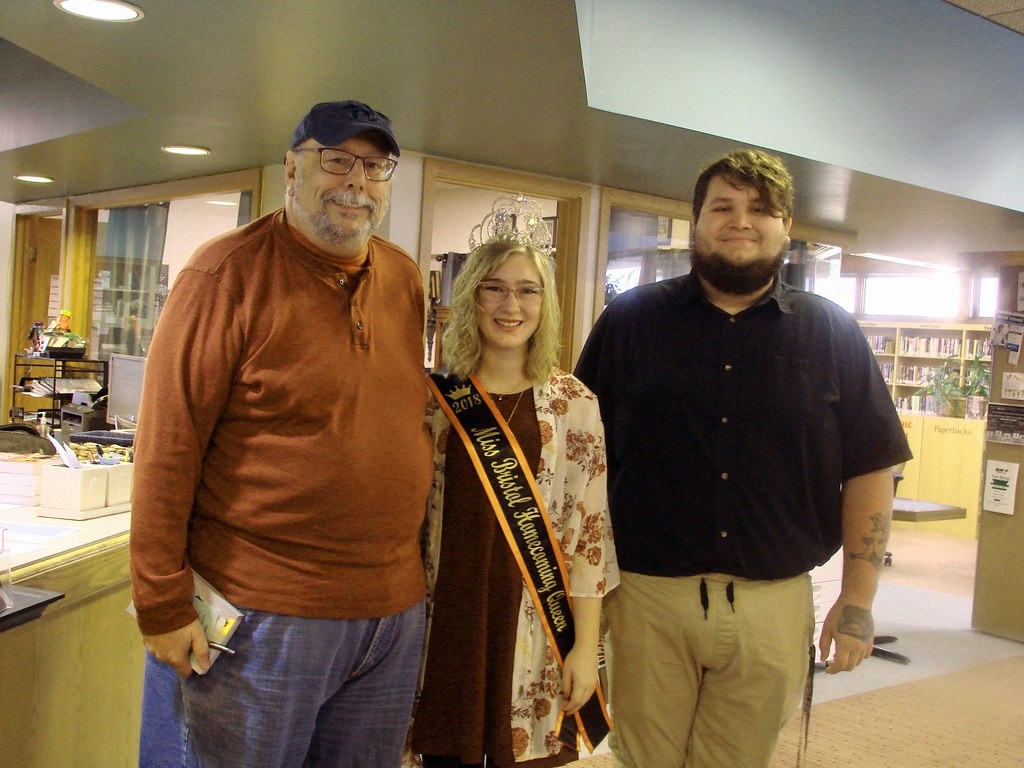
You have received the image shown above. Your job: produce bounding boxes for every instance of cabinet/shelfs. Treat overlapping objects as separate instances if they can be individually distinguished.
[855,320,992,539]
[10,354,108,437]
[89,262,169,365]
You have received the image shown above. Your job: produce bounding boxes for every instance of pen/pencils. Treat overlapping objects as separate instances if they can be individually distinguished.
[207,638,236,657]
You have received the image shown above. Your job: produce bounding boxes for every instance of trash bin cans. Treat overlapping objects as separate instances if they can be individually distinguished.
[60,402,114,451]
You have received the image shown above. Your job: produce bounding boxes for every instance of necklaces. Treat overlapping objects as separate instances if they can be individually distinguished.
[476,368,530,402]
[482,377,529,426]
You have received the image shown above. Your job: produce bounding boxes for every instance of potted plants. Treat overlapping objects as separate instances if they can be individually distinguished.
[914,350,991,422]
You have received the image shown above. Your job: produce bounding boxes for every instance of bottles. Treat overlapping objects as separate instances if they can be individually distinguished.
[58,309,72,332]
[126,314,141,356]
[32,321,46,353]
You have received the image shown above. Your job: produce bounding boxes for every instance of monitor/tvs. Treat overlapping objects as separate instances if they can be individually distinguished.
[106,353,148,429]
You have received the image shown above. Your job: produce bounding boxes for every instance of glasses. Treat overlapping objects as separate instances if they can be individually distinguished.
[477,281,544,302]
[284,147,400,183]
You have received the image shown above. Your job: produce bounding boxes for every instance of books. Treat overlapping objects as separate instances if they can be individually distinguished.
[126,566,245,675]
[864,335,993,359]
[893,395,936,412]
[878,364,938,384]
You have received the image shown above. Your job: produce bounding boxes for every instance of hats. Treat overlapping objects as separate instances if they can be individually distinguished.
[290,99,400,158]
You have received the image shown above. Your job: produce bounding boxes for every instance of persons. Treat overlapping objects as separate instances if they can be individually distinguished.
[412,235,626,768]
[569,147,918,768]
[129,99,435,768]
